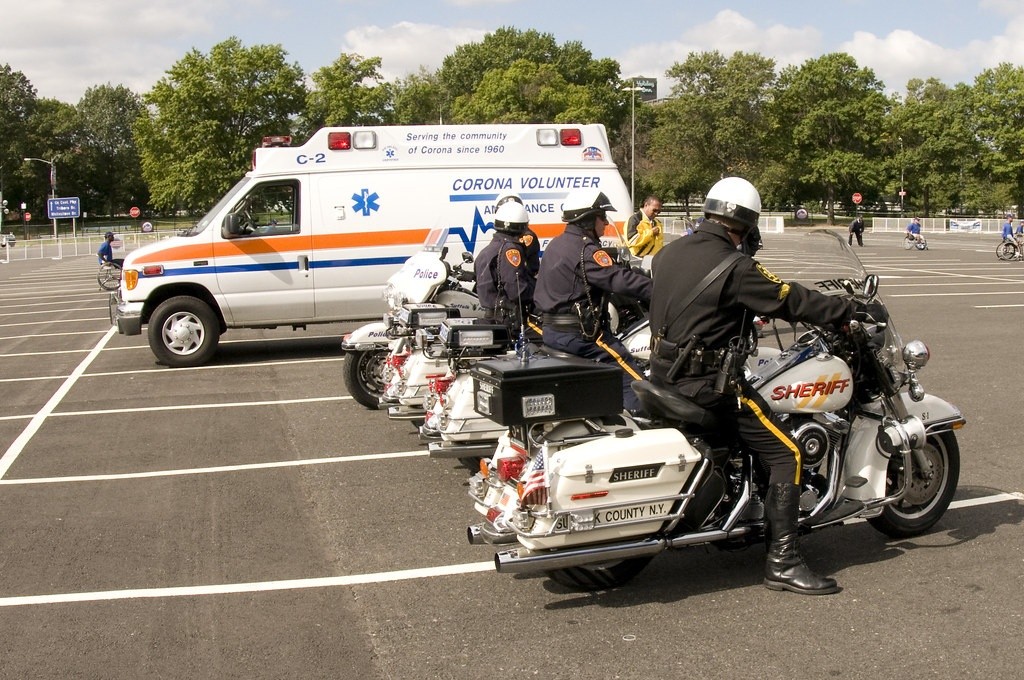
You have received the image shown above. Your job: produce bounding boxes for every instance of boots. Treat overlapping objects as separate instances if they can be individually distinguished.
[763,482,838,594]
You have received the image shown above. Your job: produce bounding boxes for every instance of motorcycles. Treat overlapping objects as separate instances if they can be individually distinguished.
[343,223,785,475]
[473,227,968,594]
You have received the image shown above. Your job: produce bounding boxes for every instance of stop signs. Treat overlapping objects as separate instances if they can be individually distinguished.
[129,207,141,219]
[852,193,863,205]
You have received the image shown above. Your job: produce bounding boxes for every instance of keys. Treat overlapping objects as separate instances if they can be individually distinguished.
[736,390,742,409]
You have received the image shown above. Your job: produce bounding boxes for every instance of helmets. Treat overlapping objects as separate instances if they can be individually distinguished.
[561,188,617,222]
[495,192,523,213]
[493,201,529,233]
[702,177,761,226]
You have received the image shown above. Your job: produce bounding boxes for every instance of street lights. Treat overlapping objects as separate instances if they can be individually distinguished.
[898,137,906,218]
[24,157,59,244]
[622,82,645,208]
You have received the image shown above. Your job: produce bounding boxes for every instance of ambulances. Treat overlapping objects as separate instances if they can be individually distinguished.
[112,123,634,370]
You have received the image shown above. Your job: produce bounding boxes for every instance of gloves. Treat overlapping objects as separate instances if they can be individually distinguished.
[852,299,888,333]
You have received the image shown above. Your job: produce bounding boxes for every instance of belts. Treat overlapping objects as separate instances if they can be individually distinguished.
[485,309,509,317]
[652,337,715,365]
[542,314,582,324]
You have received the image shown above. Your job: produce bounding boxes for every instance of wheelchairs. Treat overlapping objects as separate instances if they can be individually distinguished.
[903,232,929,251]
[95,262,122,290]
[995,236,1024,260]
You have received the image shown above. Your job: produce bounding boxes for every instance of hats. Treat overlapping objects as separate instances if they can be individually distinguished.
[915,217,921,220]
[859,215,863,221]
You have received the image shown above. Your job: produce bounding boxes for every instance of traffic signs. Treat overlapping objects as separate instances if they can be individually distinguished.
[47,197,81,219]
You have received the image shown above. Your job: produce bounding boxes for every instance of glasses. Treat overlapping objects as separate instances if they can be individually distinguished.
[588,214,607,221]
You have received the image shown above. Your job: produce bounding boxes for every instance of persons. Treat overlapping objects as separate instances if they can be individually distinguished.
[904,217,924,244]
[622,195,664,258]
[1002,215,1024,258]
[453,196,541,295]
[532,187,653,416]
[473,202,544,348]
[650,177,889,595]
[98,231,125,269]
[848,215,865,247]
